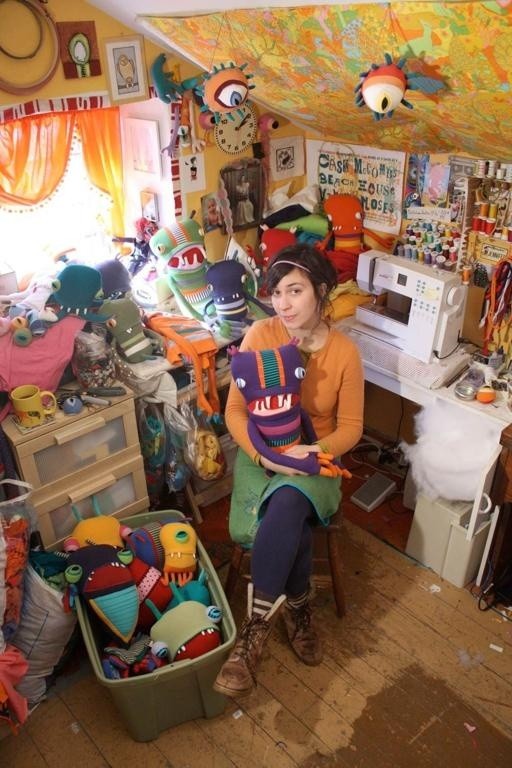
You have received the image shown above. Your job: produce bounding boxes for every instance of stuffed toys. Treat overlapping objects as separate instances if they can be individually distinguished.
[151,52,258,160]
[60,493,224,682]
[259,227,300,297]
[224,338,352,478]
[315,193,396,281]
[350,52,449,123]
[257,111,282,183]
[0,218,278,365]
[1,183,397,516]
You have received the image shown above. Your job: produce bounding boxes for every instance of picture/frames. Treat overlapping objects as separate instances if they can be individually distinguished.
[219,162,262,235]
[100,33,150,108]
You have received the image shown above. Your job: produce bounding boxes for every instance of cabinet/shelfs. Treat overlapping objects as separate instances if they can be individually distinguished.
[0,375,151,555]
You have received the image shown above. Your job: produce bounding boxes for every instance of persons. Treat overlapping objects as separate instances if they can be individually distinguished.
[211,241,369,696]
[234,174,255,227]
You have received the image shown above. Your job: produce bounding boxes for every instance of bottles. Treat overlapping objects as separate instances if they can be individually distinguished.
[11,383,57,428]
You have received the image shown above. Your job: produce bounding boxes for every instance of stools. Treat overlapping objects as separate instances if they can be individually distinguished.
[224,494,347,621]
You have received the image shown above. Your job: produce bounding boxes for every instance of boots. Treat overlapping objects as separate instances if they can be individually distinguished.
[280,597,324,668]
[211,582,287,698]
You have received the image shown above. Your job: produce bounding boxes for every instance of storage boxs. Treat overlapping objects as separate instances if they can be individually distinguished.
[73,509,237,743]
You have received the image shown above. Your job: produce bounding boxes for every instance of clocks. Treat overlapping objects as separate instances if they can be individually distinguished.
[214,102,258,156]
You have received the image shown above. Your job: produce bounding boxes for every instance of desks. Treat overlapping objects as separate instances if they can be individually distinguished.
[486,425,511,585]
[141,301,512,588]
